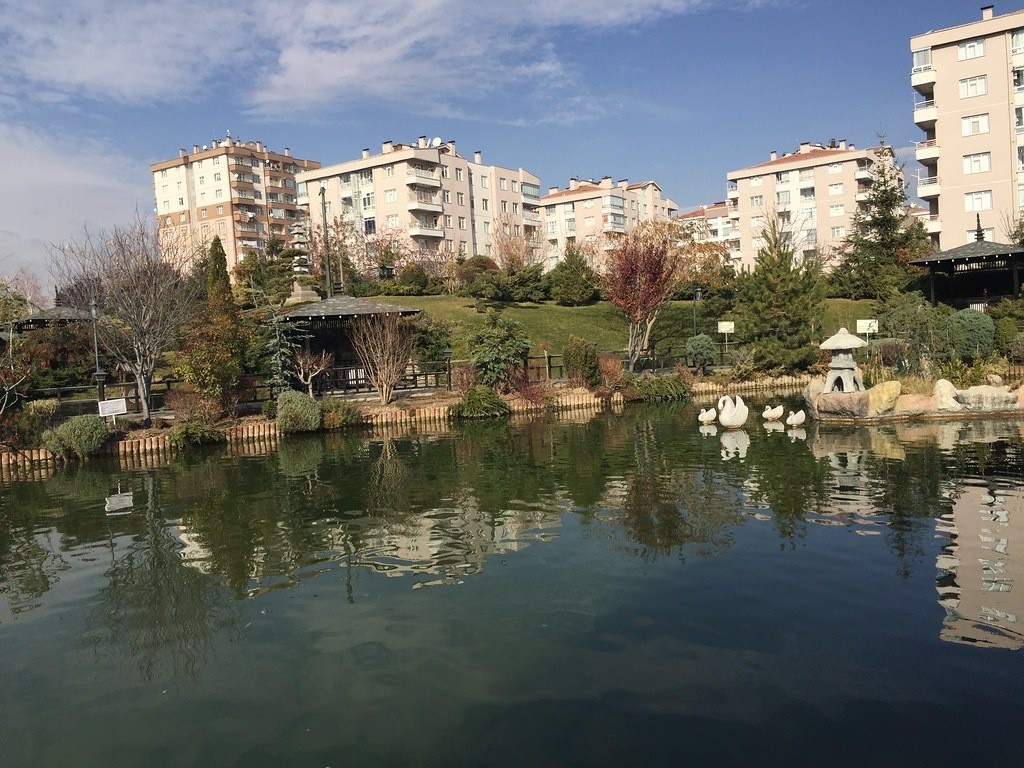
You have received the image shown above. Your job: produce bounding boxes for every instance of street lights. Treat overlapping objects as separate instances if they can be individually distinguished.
[88,300,98,372]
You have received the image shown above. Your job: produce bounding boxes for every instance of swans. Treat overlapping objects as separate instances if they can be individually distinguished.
[787,427,807,443]
[761,405,784,421]
[717,394,750,429]
[762,422,784,436]
[785,409,806,427]
[719,429,751,464]
[697,408,717,425]
[699,425,717,440]
